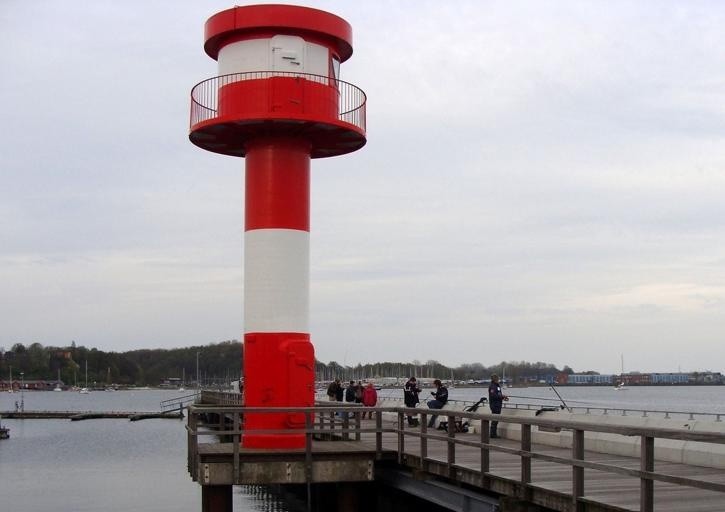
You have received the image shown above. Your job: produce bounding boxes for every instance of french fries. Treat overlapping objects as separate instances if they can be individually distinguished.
[376,377,405,405]
[393,393,432,425]
[537,369,569,412]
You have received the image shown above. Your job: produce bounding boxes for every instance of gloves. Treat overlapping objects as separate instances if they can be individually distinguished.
[80,388,93,394]
[0,421,10,440]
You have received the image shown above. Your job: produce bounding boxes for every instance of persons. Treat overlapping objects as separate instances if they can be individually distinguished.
[488,374,509,438]
[327,377,377,420]
[427,380,448,427]
[403,378,422,427]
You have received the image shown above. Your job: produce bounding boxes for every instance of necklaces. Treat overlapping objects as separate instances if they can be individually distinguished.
[615,352,630,391]
